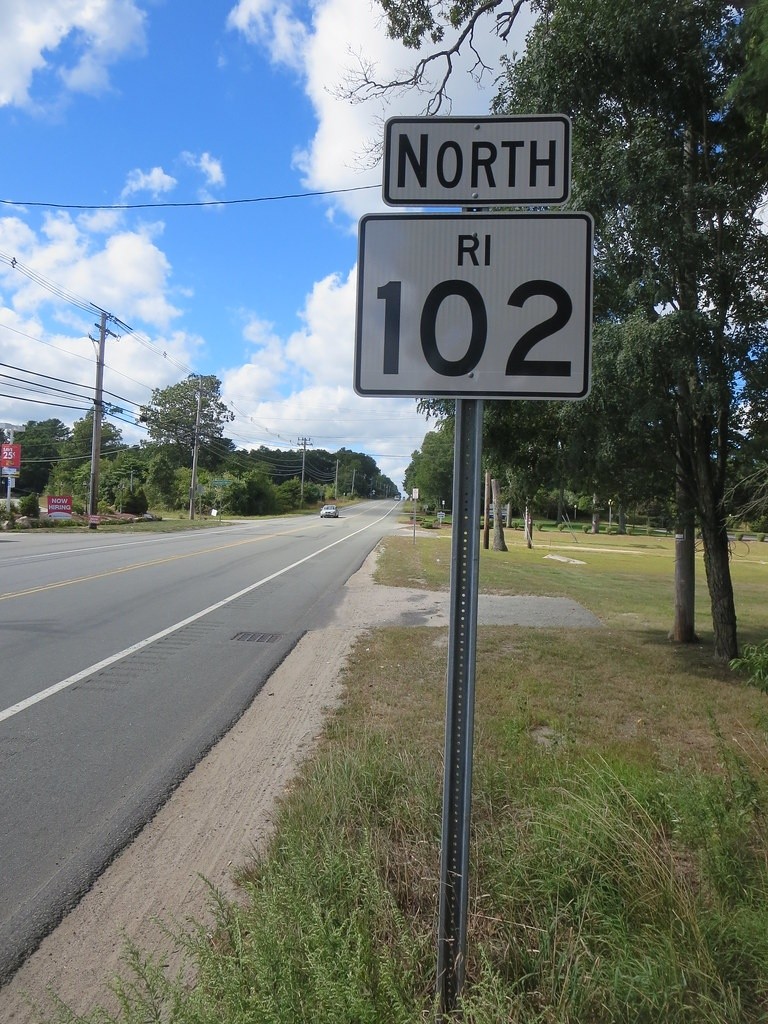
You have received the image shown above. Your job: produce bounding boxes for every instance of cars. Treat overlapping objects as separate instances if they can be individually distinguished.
[320,505,339,518]
